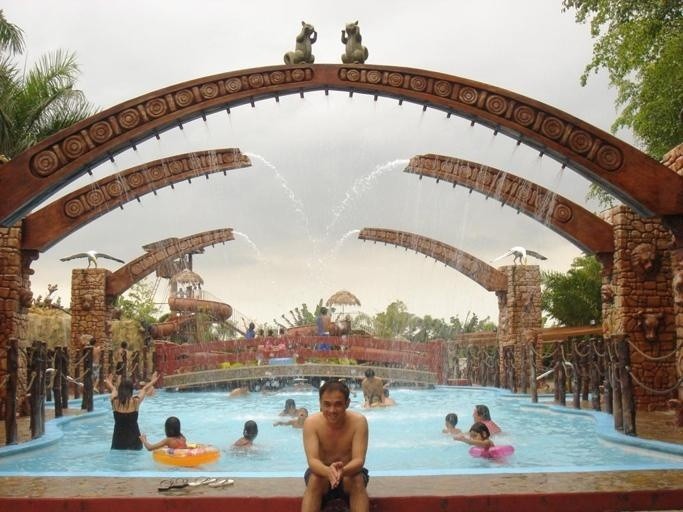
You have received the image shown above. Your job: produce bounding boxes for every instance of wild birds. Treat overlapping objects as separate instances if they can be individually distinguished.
[488,246,548,270]
[59,250,125,270]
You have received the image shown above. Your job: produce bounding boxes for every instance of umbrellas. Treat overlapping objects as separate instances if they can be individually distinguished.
[325,290,362,320]
[169,270,205,293]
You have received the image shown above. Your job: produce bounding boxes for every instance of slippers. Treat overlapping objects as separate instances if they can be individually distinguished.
[171,475,189,490]
[188,473,218,487]
[211,478,233,488]
[157,477,174,491]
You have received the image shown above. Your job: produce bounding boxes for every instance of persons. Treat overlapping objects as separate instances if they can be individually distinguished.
[174,288,184,297]
[226,381,251,397]
[470,404,506,438]
[360,369,386,407]
[232,420,258,447]
[451,421,497,459]
[257,328,266,336]
[298,380,372,512]
[101,370,160,451]
[267,330,272,336]
[84,337,102,393]
[113,341,129,388]
[243,322,256,338]
[381,387,396,405]
[136,378,156,397]
[277,398,298,416]
[137,416,187,451]
[279,328,285,335]
[440,412,463,435]
[367,393,382,407]
[315,306,331,352]
[272,407,309,429]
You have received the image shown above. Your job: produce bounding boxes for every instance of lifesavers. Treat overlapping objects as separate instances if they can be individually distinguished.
[470,445,514,458]
[229,388,249,396]
[364,396,395,407]
[153,443,220,467]
